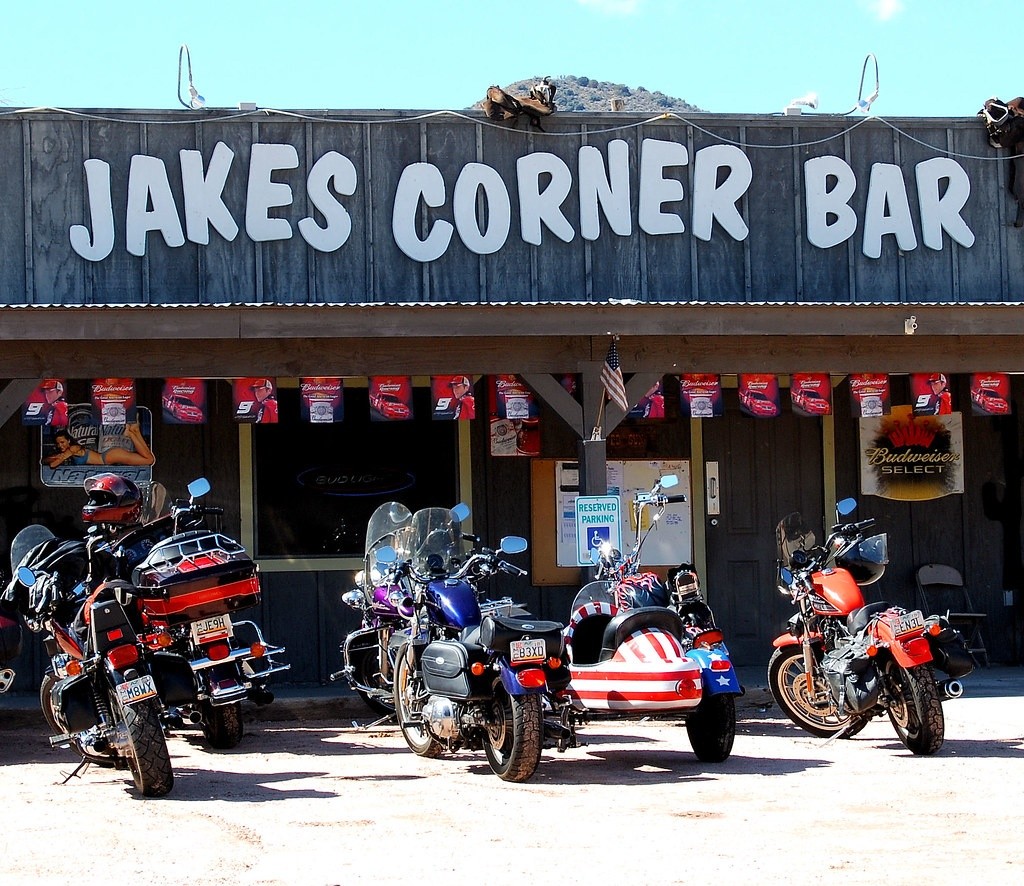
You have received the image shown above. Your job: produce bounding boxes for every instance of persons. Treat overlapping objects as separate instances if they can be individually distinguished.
[926,373,951,415]
[254,379,278,423]
[643,381,664,418]
[452,376,475,419]
[42,413,156,469]
[44,380,68,425]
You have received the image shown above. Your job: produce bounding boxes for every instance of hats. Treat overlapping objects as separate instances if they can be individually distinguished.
[450,376,470,386]
[929,373,947,383]
[253,379,272,390]
[39,380,64,392]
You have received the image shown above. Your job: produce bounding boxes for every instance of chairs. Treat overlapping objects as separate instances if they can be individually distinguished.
[599,607,684,662]
[915,563,991,669]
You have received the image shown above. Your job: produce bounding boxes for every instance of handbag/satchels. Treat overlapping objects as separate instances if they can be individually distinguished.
[480,616,564,657]
[923,618,974,678]
[420,641,493,699]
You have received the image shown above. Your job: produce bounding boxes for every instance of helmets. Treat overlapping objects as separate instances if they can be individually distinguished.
[835,532,885,585]
[81,473,144,526]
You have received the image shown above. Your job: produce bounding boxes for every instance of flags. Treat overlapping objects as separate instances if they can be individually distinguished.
[600,339,629,413]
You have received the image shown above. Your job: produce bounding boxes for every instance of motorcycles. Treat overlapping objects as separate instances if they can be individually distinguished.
[767,497,966,758]
[0,523,199,796]
[564,473,747,766]
[79,479,294,752]
[329,500,577,783]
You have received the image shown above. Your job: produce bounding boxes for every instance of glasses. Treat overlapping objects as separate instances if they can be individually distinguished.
[449,383,465,388]
[251,386,266,391]
[42,389,58,393]
[927,380,941,384]
